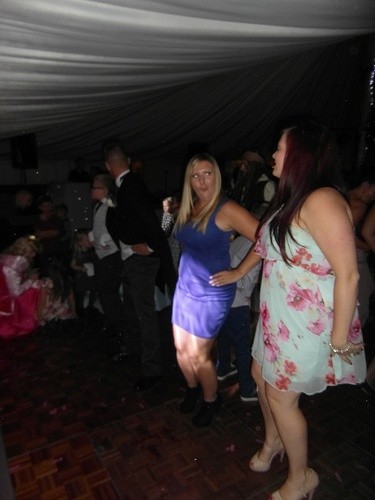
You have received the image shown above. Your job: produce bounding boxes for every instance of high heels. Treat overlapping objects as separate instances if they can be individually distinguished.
[269,468,319,500]
[249,443,285,472]
[192,391,222,426]
[179,383,202,415]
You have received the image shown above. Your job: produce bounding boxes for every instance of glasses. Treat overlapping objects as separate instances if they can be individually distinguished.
[90,186,105,190]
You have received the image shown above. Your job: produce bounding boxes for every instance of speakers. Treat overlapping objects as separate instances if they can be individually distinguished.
[10,133,38,169]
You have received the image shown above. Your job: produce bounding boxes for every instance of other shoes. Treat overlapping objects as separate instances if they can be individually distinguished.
[216,364,238,380]
[239,391,259,402]
[113,351,132,363]
[102,333,123,357]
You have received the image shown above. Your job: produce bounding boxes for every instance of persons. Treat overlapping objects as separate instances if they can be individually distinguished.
[0,190,95,339]
[247,126,375,500]
[77,141,278,428]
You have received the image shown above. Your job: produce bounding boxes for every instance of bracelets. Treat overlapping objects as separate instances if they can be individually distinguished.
[329,342,351,352]
